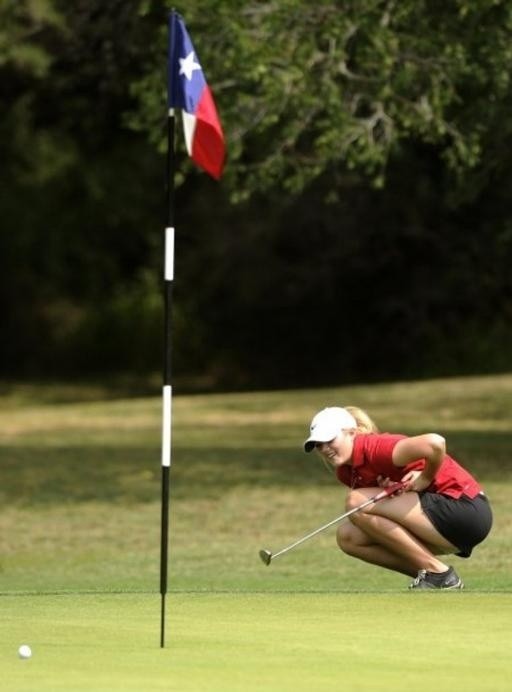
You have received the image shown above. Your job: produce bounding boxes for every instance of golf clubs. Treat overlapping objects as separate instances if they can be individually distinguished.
[259,479,410,565]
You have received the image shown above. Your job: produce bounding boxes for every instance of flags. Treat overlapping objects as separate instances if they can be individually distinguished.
[166,9,225,182]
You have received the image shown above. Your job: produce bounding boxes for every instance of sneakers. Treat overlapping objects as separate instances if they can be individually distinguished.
[408,565,461,590]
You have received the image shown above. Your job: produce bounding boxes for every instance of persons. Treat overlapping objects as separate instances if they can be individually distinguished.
[301,404,494,591]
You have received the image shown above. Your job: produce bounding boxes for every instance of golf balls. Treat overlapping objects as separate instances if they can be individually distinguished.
[20,645,31,658]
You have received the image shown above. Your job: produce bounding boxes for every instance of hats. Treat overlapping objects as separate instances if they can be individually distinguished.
[304,407,357,453]
